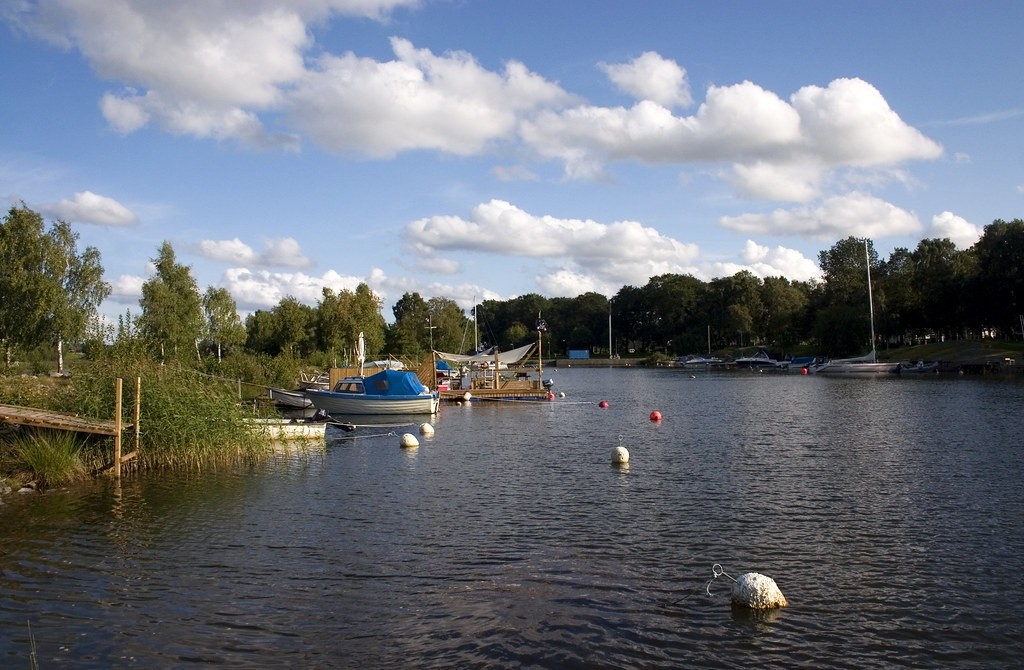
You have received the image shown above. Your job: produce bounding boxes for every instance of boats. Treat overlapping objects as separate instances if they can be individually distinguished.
[652,351,821,369]
[306,370,441,415]
[264,372,330,408]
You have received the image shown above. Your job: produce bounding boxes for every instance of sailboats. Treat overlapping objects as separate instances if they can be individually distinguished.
[816,240,903,372]
